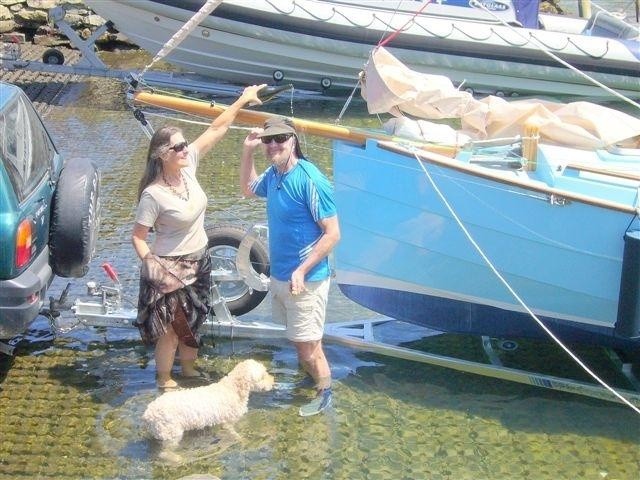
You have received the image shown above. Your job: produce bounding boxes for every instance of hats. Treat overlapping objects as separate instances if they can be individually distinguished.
[257,123,299,139]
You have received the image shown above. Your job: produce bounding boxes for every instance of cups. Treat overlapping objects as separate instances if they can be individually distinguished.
[520,135,540,172]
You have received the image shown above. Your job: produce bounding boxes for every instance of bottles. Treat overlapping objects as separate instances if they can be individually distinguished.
[242,84,292,108]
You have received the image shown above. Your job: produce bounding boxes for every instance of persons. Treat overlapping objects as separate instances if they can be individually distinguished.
[131,82,270,391]
[240,120,341,418]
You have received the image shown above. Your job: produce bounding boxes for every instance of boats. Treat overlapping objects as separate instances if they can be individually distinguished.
[81,0,640,104]
[129,0,640,348]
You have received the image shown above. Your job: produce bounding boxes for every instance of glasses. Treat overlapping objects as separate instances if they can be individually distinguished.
[260,132,293,144]
[169,139,188,153]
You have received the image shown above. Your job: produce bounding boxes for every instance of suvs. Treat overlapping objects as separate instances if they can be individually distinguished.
[1,75,105,357]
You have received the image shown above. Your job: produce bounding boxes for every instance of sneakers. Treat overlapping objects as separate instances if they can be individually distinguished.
[297,387,332,417]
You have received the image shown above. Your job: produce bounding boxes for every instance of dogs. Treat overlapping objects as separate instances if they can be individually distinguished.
[142,358,275,462]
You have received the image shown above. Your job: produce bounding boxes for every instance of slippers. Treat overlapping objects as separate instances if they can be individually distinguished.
[158,383,182,393]
[184,371,212,380]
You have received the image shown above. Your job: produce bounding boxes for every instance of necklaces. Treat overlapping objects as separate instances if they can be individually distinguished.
[162,169,190,203]
